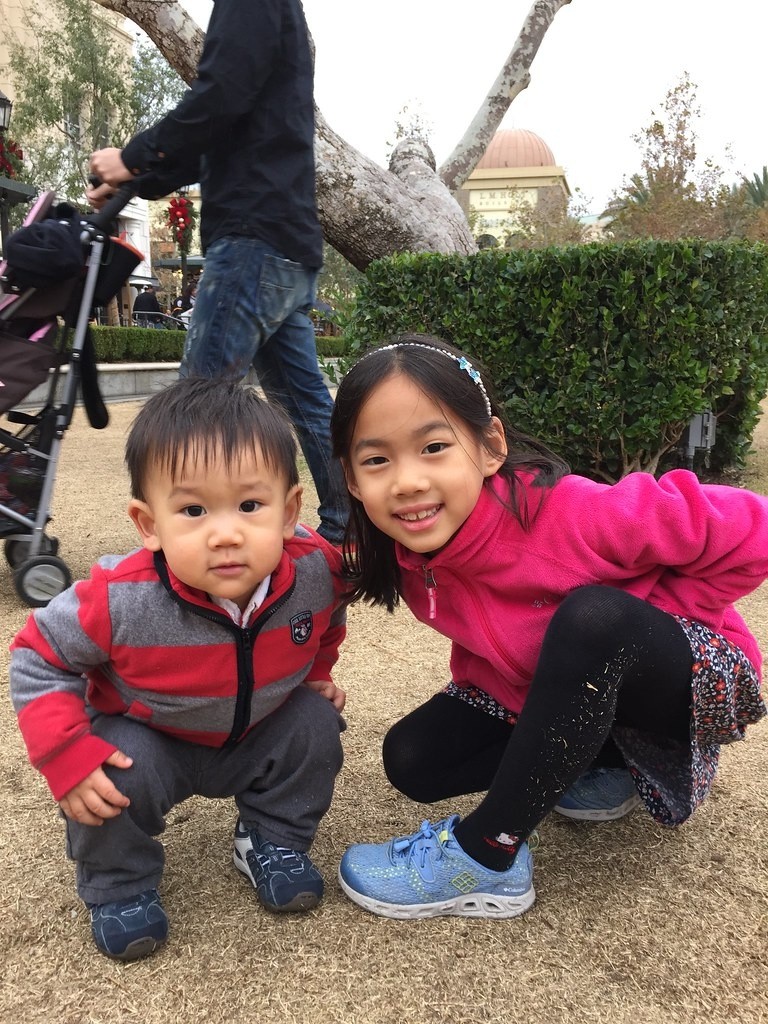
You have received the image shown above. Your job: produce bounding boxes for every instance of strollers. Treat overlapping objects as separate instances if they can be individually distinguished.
[0,168,148,611]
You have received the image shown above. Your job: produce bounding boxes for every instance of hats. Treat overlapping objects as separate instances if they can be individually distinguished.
[144,285,153,288]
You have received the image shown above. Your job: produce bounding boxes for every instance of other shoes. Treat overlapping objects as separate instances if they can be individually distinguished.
[328,541,360,564]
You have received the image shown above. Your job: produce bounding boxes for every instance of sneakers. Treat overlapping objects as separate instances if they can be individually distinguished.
[81,887,169,961]
[232,817,325,912]
[552,735,643,821]
[336,814,536,920]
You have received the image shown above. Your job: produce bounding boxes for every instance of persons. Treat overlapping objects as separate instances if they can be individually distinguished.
[7,378,349,959]
[330,336,768,922]
[132,283,161,323]
[173,282,197,318]
[85,0,355,550]
